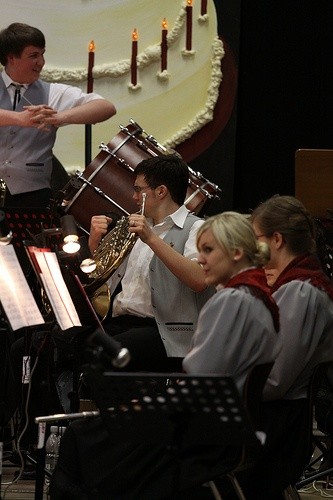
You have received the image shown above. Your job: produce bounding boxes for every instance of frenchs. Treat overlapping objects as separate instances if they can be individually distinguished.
[82,192,146,300]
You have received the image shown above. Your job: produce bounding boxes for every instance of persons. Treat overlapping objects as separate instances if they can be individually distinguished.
[48,212,284,500]
[1,22,117,207]
[14,152,218,481]
[249,196,333,403]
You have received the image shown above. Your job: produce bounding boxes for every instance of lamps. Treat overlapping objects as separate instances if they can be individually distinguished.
[59,215,81,254]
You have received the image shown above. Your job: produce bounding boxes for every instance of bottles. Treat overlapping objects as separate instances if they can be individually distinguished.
[45,426,67,486]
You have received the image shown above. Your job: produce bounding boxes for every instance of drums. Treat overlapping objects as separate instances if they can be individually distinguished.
[55,117,222,241]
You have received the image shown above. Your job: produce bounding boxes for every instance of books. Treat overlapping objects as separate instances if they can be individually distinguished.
[34,251,83,331]
[0,245,46,331]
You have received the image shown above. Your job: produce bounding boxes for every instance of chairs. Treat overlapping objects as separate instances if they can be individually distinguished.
[207,360,277,500]
